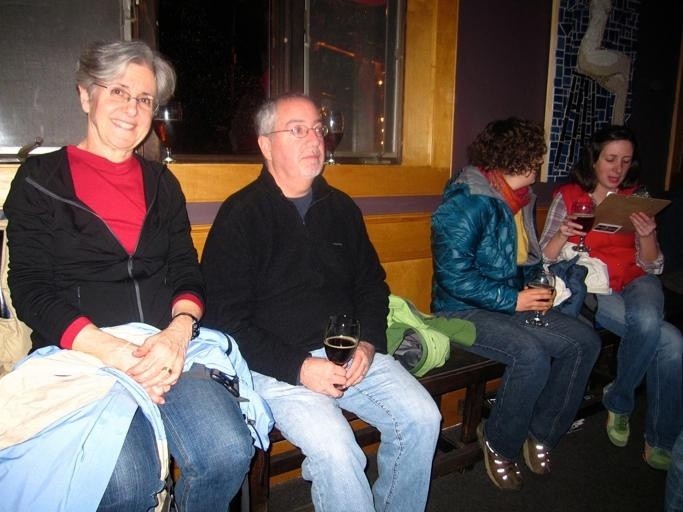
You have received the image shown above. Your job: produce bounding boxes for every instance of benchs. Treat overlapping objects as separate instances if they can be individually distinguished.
[0,164,683,512]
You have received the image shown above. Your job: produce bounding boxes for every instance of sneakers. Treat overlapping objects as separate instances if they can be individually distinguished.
[524,438,553,474]
[645,441,671,470]
[476,419,524,491]
[603,382,630,448]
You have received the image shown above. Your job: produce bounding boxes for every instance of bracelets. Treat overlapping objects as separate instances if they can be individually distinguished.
[166,312,200,341]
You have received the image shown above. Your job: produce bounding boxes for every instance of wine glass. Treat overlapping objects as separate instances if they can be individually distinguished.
[319,107,345,166]
[152,99,184,163]
[570,198,596,254]
[522,268,556,328]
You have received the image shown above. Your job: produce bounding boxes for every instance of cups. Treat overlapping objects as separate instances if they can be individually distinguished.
[323,313,361,366]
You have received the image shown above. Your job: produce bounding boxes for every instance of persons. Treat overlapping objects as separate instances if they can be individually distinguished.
[201,90,446,512]
[5,39,259,512]
[540,123,683,512]
[428,112,603,498]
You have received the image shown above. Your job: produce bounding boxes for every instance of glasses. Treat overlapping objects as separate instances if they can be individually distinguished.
[92,81,159,112]
[262,124,329,137]
